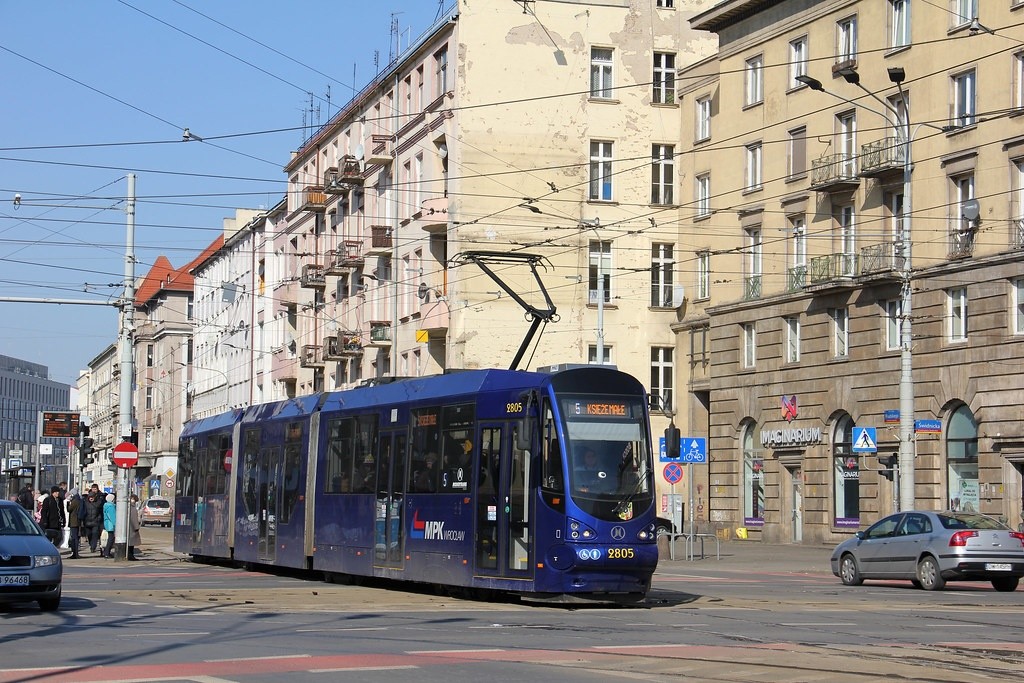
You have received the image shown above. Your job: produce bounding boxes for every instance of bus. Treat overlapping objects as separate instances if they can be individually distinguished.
[174,250,681,605]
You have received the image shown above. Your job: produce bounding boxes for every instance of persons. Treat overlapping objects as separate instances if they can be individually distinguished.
[40,486,66,547]
[102,494,116,558]
[354,459,374,492]
[59,482,67,498]
[60,492,71,549]
[574,449,601,471]
[196,497,206,540]
[88,484,107,549]
[128,494,141,561]
[37,488,50,511]
[19,483,34,510]
[416,451,437,492]
[368,462,387,499]
[67,487,82,558]
[84,492,102,552]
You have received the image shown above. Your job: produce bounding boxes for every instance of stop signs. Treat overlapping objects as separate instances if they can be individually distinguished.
[224,449,232,472]
[112,443,138,467]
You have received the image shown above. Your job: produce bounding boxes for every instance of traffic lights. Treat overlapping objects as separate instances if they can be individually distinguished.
[877,456,892,481]
[84,438,95,467]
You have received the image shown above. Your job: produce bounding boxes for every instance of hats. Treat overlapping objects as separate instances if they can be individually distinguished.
[51,486,60,493]
[106,493,115,502]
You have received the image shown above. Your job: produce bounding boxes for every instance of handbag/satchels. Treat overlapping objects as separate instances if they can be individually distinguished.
[100,529,109,547]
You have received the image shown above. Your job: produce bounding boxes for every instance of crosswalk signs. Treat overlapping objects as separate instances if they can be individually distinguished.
[851,427,877,452]
[150,481,160,490]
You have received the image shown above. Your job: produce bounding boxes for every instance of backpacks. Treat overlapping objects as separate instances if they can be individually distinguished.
[74,498,87,520]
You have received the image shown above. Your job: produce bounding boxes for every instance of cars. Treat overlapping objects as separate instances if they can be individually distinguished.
[831,510,1024,592]
[0,500,63,613]
[137,495,173,528]
[656,517,678,541]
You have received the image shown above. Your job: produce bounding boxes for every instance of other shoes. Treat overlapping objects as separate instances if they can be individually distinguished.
[104,551,112,558]
[128,556,140,561]
[91,547,95,553]
[67,554,80,559]
